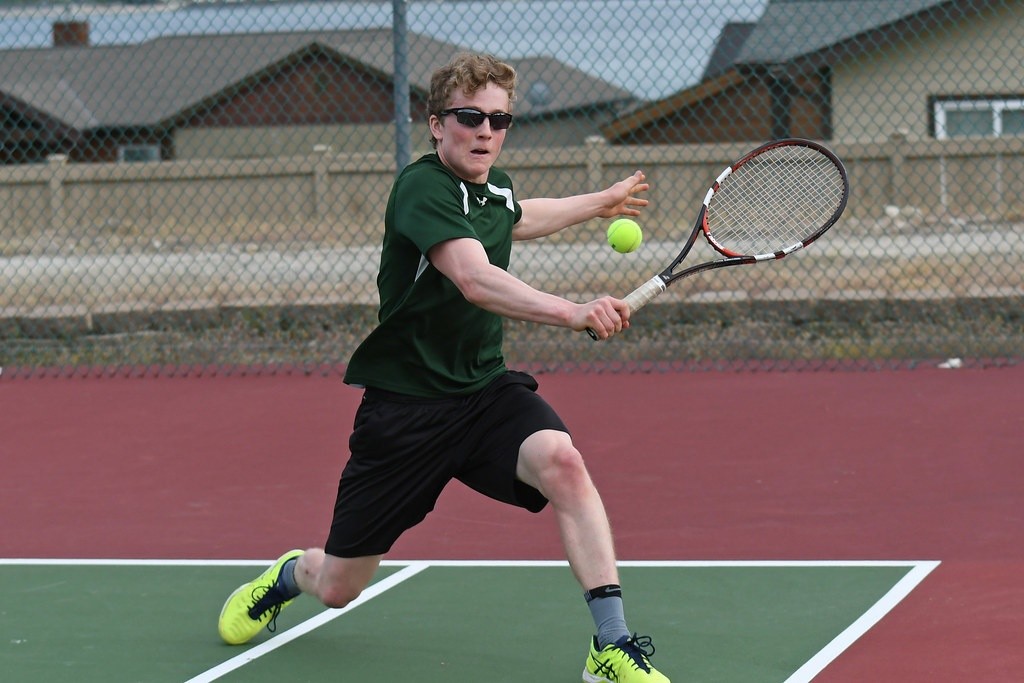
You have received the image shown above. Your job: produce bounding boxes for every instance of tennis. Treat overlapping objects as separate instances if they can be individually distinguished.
[606,218,643,254]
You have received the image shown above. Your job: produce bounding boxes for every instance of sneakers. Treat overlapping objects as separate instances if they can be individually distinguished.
[218,548,306,645]
[582,631,672,683]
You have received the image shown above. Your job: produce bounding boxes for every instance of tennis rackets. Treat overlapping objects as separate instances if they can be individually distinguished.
[584,137,851,341]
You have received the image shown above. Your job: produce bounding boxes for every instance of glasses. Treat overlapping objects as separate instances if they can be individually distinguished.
[439,108,513,130]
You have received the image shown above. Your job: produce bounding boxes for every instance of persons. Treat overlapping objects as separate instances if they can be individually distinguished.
[217,54,677,683]
